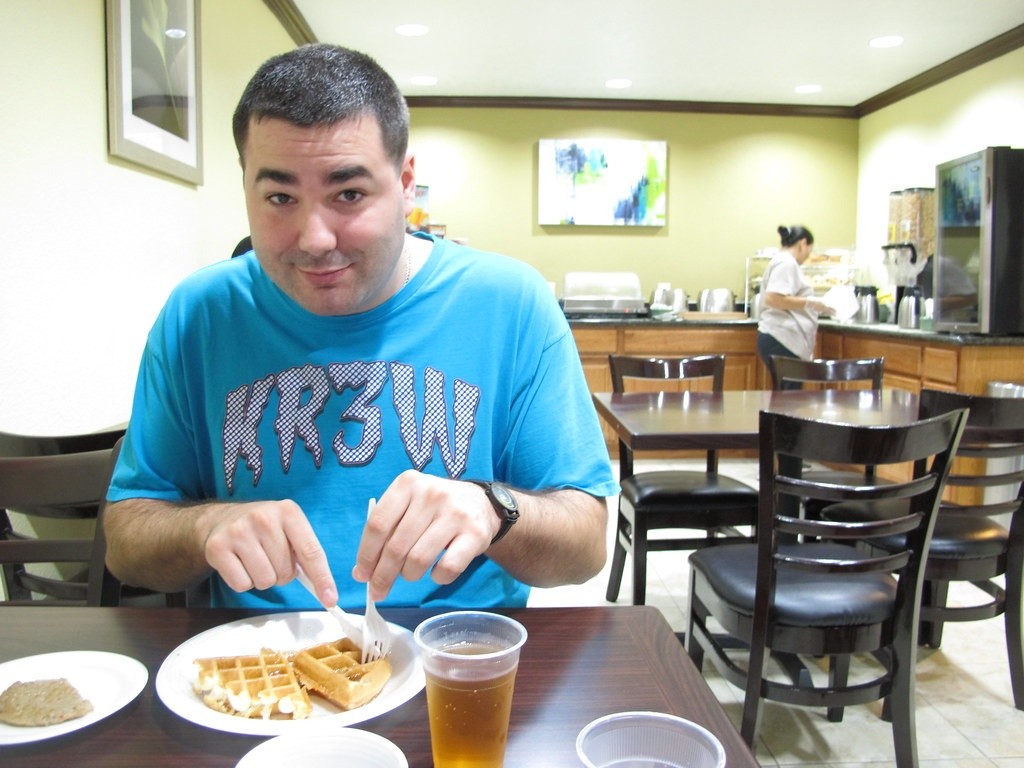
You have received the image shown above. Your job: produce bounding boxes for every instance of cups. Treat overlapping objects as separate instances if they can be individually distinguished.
[234,728,408,768]
[576,711,726,768]
[414,611,527,768]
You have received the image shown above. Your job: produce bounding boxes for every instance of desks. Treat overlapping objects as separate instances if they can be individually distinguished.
[591,389,921,689]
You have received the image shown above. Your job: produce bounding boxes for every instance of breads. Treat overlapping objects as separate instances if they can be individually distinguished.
[190,647,311,721]
[291,635,392,710]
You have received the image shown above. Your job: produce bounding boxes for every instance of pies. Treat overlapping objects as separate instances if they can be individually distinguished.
[0,678,92,726]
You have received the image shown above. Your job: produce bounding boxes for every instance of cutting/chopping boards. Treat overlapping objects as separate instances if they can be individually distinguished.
[680,312,746,321]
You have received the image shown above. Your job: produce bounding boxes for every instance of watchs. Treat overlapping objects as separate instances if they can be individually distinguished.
[461,480,520,545]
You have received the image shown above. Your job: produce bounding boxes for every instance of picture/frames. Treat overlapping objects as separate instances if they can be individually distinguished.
[103,0,203,186]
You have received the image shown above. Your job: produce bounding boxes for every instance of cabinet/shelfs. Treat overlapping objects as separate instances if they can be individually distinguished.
[623,328,762,462]
[921,342,1024,508]
[572,326,622,460]
[841,336,923,485]
[821,332,842,361]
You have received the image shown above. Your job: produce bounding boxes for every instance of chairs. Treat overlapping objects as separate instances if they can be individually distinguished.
[2,427,190,607]
[607,349,760,604]
[686,406,972,768]
[817,386,1024,722]
[769,353,897,545]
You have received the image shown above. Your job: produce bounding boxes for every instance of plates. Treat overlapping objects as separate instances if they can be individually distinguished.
[156,612,426,735]
[0,651,148,747]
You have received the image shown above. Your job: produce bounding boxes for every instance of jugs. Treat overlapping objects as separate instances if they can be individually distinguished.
[854,287,879,323]
[898,286,925,329]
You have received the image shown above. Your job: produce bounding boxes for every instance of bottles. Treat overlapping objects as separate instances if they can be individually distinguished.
[406,184,432,235]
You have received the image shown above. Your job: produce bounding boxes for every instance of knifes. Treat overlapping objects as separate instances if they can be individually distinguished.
[295,562,381,656]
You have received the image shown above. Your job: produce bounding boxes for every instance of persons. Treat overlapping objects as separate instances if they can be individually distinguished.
[102,45,623,607]
[756,225,836,482]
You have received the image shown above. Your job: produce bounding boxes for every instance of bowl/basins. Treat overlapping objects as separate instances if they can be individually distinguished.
[706,289,736,311]
[651,309,674,321]
[697,289,710,312]
[666,288,689,313]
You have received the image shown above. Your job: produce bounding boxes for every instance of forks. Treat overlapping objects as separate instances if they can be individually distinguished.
[362,498,390,664]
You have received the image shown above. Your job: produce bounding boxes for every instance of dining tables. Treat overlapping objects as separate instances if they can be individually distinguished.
[0,605,760,768]
